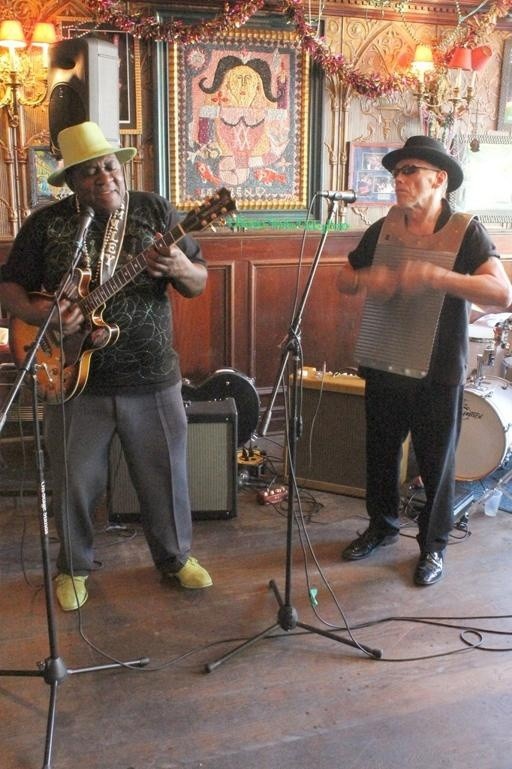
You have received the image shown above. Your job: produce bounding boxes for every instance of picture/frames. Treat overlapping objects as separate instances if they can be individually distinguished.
[447,132,511,226]
[52,14,144,136]
[492,36,512,133]
[345,138,404,210]
[26,144,75,210]
[165,24,315,215]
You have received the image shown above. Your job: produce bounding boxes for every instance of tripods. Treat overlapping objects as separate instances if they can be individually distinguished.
[0,261,150,769]
[205,203,382,677]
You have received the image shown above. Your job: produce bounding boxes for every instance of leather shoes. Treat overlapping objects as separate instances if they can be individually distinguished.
[166,555,214,590]
[54,572,89,613]
[412,548,445,587]
[341,527,401,561]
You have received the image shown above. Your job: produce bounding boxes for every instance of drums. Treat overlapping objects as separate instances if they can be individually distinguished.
[467,323,496,369]
[455,375,512,481]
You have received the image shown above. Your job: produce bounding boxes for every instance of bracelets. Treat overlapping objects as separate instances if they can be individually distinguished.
[352,272,359,294]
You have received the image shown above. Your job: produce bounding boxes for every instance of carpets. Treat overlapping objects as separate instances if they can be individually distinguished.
[403,445,512,513]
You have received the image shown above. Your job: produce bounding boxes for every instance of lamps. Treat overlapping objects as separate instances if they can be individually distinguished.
[411,44,493,130]
[0,18,65,112]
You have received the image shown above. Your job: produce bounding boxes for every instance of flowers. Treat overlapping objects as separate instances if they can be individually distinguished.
[86,0,509,101]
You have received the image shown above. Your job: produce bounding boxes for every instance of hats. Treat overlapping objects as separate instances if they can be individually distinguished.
[47,119,139,188]
[380,135,465,194]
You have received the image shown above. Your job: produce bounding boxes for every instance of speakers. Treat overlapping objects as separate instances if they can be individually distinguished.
[285,367,411,501]
[108,397,237,523]
[48,38,120,154]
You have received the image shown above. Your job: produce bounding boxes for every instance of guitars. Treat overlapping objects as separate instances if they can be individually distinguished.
[9,188,237,406]
[182,368,261,448]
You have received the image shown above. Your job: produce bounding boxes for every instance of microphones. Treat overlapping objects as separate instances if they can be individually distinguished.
[316,189,357,203]
[71,206,95,260]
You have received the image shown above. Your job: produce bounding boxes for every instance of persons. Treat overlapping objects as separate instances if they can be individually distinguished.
[1,117,213,613]
[330,132,511,589]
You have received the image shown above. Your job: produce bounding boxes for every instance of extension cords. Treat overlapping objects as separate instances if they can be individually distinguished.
[237,449,266,476]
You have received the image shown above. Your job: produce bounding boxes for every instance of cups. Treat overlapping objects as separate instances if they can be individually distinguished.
[0,327,10,346]
[482,489,503,517]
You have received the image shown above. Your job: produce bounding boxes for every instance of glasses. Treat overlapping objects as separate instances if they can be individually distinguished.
[390,164,440,179]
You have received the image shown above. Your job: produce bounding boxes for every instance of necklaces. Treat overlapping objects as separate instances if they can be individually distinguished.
[73,194,119,284]
